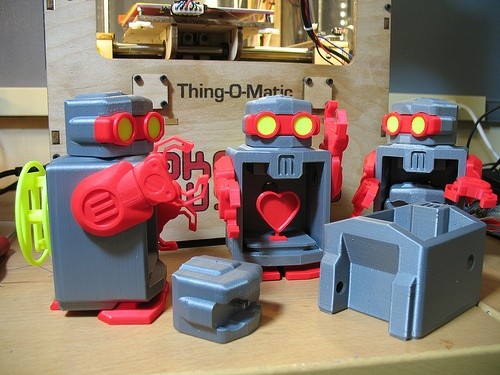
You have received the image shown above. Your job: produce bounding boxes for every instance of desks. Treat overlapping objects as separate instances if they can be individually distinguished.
[1,219,500,375]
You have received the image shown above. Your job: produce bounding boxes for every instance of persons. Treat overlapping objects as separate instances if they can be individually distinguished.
[348,94,499,231]
[210,82,351,282]
[14,90,209,327]
